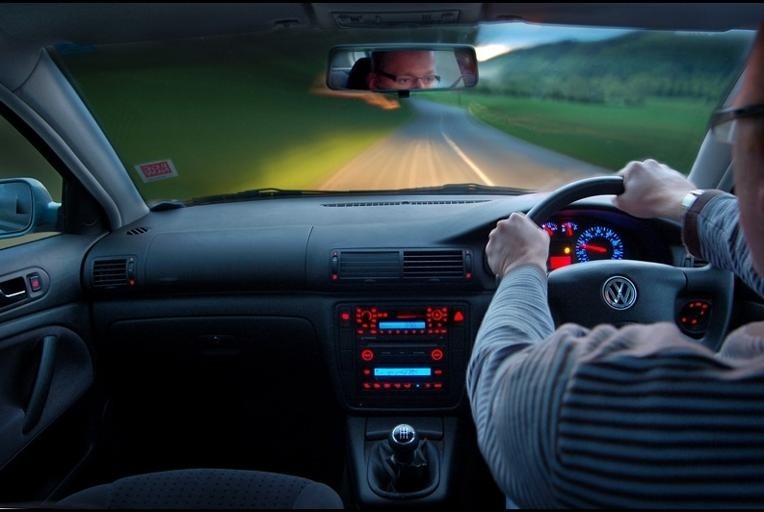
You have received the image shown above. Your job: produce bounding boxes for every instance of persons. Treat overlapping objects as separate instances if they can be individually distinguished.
[368,48,435,89]
[466,17,763,510]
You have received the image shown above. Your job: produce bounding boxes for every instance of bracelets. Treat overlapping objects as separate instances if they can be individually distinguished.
[680,188,708,224]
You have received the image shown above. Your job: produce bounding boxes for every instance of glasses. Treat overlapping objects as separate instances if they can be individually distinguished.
[709,104,763,144]
[381,72,441,86]
